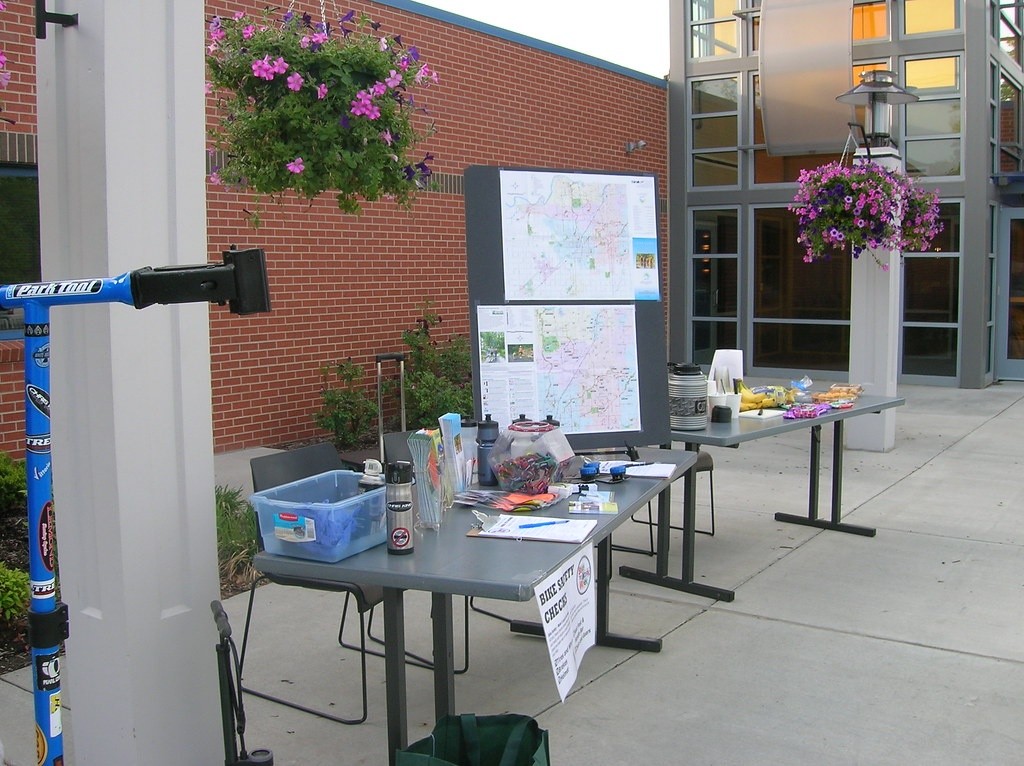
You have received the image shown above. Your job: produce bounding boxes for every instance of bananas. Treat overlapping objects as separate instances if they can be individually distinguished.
[734,379,778,411]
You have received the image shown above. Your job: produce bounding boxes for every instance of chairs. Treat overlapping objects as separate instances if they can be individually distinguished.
[374,429,510,674]
[237,442,433,724]
[613,449,716,554]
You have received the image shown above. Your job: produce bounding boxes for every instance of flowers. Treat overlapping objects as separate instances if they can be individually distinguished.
[205,6,438,228]
[792,157,945,270]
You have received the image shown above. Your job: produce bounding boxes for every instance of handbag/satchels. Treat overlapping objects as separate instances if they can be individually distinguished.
[395,713,551,766]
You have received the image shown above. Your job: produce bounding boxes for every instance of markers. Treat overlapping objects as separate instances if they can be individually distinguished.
[733,378,738,394]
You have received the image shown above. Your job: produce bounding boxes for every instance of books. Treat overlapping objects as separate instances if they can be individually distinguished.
[569,500,619,514]
[740,409,786,419]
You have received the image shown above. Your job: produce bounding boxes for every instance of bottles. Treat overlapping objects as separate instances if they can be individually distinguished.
[461,413,478,474]
[477,413,501,486]
[384,460,417,555]
[512,414,560,426]
[349,458,385,541]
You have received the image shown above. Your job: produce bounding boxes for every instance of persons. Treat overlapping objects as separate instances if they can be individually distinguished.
[487,346,498,359]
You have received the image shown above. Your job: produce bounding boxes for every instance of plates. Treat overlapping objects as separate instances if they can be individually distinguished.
[738,409,786,419]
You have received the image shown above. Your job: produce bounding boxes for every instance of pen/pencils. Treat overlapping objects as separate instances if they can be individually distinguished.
[720,379,726,394]
[623,462,655,467]
[518,520,570,530]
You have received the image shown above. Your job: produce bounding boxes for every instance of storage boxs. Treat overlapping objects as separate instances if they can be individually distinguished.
[249,470,386,563]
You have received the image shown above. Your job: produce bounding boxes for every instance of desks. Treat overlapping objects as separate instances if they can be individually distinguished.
[251,448,698,766]
[619,376,904,602]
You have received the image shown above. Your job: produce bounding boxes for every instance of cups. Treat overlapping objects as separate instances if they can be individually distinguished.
[708,393,742,418]
